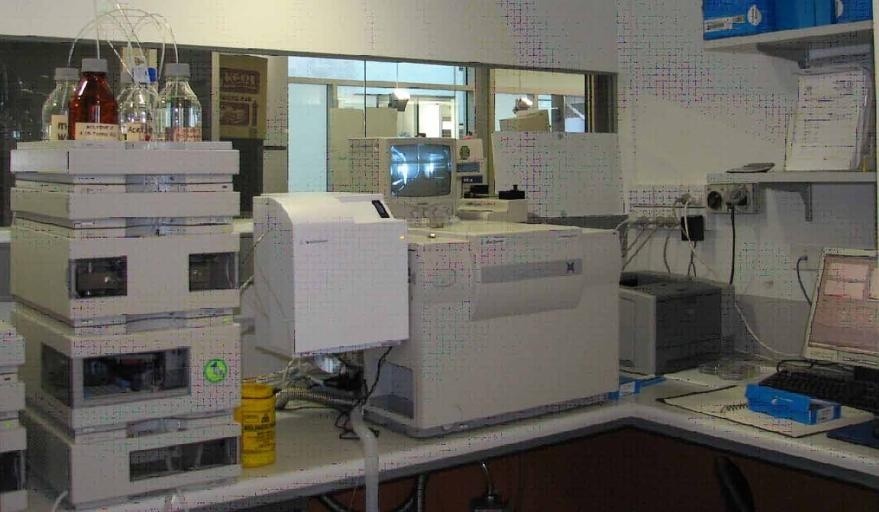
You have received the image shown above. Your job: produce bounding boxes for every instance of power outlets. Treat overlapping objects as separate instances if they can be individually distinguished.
[629,184,761,230]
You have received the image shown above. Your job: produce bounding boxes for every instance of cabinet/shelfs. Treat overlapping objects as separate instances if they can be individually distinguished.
[703,21,877,221]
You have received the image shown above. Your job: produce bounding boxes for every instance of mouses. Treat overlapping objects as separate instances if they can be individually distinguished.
[872,421,879,439]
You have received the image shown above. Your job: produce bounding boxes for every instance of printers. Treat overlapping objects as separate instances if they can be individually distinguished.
[618,270,736,375]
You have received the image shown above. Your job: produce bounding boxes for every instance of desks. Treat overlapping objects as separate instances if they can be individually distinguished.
[1,364,878,511]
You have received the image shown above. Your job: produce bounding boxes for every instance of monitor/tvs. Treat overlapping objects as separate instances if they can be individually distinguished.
[801,247,879,381]
[380,138,457,205]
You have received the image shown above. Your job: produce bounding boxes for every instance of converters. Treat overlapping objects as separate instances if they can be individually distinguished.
[681,214,706,242]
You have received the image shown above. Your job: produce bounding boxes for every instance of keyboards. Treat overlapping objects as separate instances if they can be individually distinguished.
[759,368,879,415]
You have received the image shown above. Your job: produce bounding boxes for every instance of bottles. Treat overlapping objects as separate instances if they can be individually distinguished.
[42,58,203,144]
[236,380,276,469]
